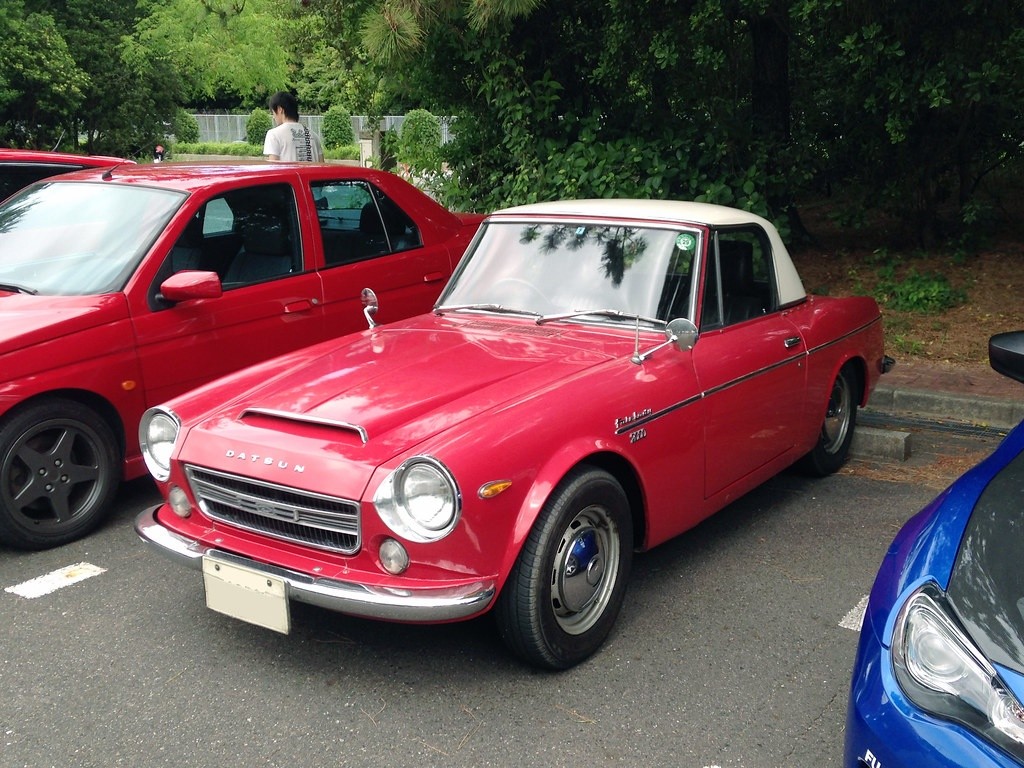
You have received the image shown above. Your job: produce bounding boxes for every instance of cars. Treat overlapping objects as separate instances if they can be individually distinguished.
[1,158,491,551]
[135,198,896,675]
[841,330,1024,768]
[0,147,138,204]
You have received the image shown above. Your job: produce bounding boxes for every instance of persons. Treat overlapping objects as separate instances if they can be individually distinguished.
[263,91,330,210]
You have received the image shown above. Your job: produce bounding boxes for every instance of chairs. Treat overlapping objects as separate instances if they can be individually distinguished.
[151,216,210,305]
[675,238,754,330]
[222,210,293,292]
